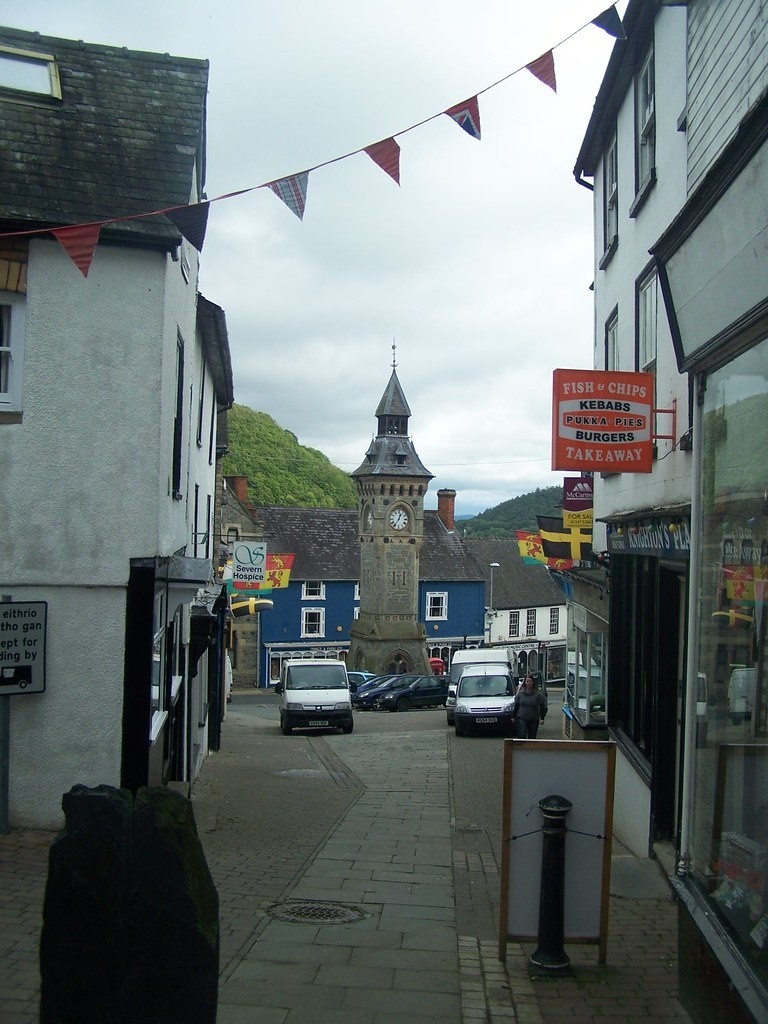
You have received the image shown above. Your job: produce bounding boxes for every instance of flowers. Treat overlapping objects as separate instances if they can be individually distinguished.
[714,860,764,890]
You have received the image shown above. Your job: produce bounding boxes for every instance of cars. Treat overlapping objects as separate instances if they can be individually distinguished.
[379,675,449,712]
[348,672,377,684]
[357,675,395,693]
[352,675,439,711]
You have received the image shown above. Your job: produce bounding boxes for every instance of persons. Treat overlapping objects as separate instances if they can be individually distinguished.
[547,663,566,687]
[512,675,546,739]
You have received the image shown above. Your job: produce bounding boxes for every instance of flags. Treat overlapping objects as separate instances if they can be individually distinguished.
[222,554,294,594]
[515,530,573,569]
[231,597,273,617]
[713,566,768,631]
[537,515,599,561]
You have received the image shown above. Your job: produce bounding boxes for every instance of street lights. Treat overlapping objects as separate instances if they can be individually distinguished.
[489,563,500,643]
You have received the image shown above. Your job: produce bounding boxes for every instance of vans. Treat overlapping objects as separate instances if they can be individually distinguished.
[567,664,602,710]
[726,668,755,726]
[445,648,520,727]
[695,673,715,741]
[448,664,548,737]
[275,659,357,736]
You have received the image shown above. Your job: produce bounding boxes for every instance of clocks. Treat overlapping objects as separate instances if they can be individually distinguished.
[389,507,410,531]
[367,510,373,526]
